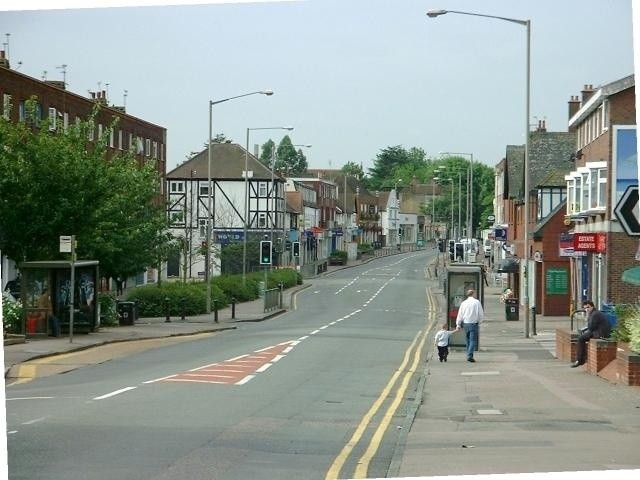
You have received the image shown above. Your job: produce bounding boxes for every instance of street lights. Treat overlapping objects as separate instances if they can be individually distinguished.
[427,8,532,338]
[393,177,403,248]
[205,90,275,312]
[271,143,312,276]
[433,149,473,264]
[241,127,296,285]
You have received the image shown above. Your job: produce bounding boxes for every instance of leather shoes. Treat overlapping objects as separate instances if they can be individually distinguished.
[573,360,583,367]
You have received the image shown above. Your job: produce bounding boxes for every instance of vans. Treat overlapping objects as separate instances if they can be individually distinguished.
[482,237,491,258]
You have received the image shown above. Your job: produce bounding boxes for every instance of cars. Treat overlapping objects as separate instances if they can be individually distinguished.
[4,278,42,304]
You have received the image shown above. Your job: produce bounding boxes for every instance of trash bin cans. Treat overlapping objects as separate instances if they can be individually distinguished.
[504,298,519,321]
[118,301,135,325]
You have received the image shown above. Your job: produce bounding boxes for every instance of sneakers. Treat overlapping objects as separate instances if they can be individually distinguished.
[467,356,475,362]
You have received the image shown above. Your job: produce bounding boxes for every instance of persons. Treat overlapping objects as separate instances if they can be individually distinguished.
[569,299,612,369]
[432,322,460,363]
[36,288,63,338]
[455,289,485,362]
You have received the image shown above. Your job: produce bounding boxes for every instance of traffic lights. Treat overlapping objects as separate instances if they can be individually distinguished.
[284,239,292,252]
[260,241,273,265]
[292,242,301,257]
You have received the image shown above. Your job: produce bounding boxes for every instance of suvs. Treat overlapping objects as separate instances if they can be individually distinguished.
[459,238,480,257]
